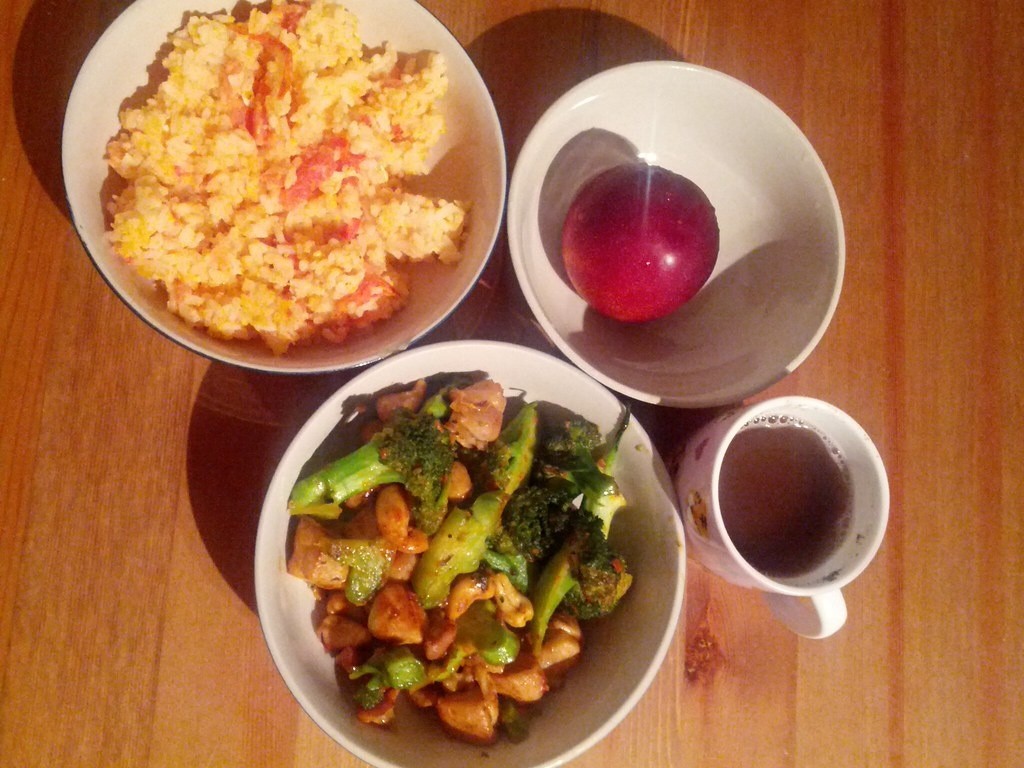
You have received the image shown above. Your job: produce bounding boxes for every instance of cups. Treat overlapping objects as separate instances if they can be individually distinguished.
[673,394,890,639]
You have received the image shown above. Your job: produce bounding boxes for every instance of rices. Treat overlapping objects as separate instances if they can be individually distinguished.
[101,0,473,353]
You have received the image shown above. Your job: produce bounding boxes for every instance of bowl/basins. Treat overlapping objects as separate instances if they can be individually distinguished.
[504,60,850,412]
[61,0,508,373]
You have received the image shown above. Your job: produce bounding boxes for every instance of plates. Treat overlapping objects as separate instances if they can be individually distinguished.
[254,339,686,768]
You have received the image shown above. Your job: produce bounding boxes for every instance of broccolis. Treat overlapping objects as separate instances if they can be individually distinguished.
[287,385,634,746]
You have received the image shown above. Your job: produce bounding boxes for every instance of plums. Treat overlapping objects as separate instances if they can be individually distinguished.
[562,164,718,321]
